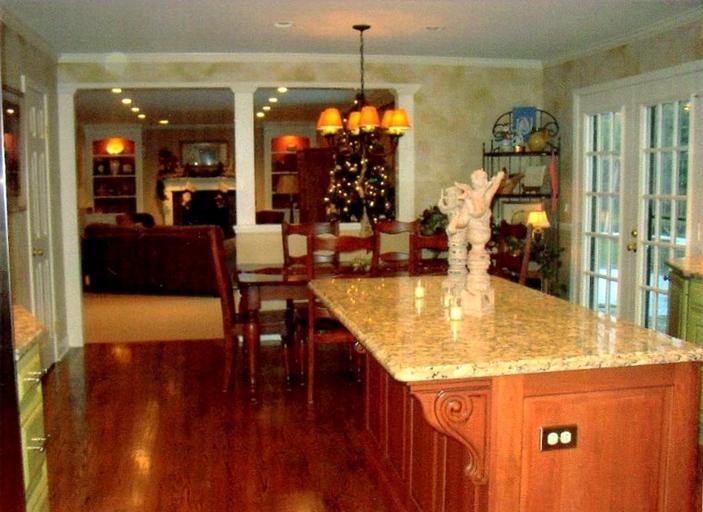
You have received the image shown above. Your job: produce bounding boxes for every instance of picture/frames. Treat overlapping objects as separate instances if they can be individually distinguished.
[499,200,545,229]
[174,137,233,170]
[0,82,27,214]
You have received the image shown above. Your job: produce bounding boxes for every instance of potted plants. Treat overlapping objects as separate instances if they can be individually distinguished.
[504,231,566,297]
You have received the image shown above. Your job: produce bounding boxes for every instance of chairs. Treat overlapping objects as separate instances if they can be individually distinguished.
[294,229,384,404]
[279,221,343,274]
[374,215,424,281]
[405,230,465,278]
[205,224,294,396]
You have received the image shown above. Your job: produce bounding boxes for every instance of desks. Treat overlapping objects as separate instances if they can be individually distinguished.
[229,258,447,386]
[304,275,702,512]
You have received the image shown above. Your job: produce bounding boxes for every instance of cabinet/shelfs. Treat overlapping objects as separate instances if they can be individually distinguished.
[12,320,49,510]
[476,106,566,296]
[663,256,703,445]
[264,120,318,222]
[84,124,141,221]
[294,146,341,222]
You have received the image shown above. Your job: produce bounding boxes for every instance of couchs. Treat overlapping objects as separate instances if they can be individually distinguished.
[76,222,232,295]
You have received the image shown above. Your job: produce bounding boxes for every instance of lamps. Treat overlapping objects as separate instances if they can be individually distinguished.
[316,24,411,138]
[510,209,553,287]
[274,174,300,223]
